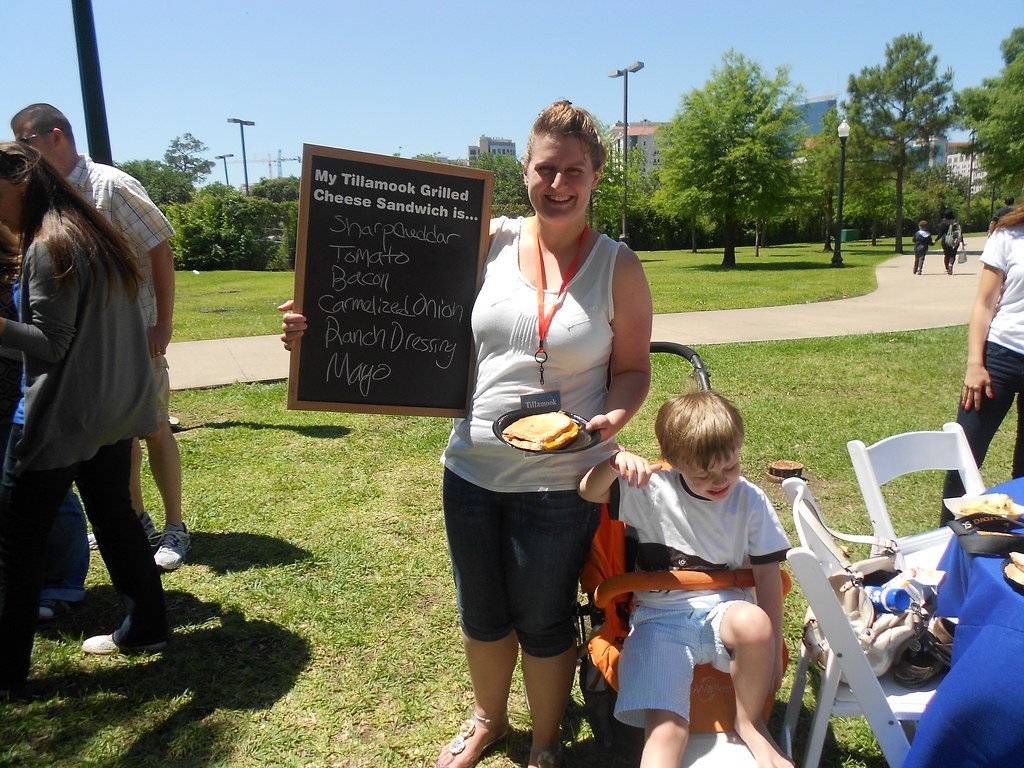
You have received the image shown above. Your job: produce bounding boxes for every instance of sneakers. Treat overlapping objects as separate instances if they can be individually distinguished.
[88,512,157,550]
[82,630,166,655]
[155,523,192,572]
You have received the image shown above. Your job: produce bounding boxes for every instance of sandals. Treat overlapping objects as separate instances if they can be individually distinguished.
[527,743,564,768]
[434,712,510,768]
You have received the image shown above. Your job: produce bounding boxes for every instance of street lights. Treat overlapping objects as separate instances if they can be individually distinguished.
[608,60,644,245]
[227,118,256,196]
[830,118,851,267]
[215,154,234,186]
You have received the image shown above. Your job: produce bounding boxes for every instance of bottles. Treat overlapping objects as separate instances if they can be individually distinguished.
[863,586,911,614]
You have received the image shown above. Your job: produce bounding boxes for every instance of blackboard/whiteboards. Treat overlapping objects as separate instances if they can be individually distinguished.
[286,143,496,415]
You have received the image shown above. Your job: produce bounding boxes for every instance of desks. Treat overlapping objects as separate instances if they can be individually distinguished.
[893,476,1024,768]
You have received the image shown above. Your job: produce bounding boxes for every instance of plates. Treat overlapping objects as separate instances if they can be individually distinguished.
[492,410,601,455]
[942,493,1024,521]
[1000,553,1024,591]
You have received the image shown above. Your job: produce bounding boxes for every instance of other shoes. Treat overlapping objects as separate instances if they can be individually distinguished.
[913,268,923,275]
[945,264,953,275]
[0,679,32,701]
[39,599,68,618]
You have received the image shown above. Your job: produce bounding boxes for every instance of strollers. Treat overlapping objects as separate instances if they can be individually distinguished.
[575,342,793,768]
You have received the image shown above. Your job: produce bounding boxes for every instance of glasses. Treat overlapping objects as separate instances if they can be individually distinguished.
[17,133,41,143]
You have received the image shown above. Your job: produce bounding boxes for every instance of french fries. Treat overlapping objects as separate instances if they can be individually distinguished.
[958,493,1020,516]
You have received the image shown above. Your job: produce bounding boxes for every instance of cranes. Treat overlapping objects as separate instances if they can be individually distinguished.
[228,149,301,177]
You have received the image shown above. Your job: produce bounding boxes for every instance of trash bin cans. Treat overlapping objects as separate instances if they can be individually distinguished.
[841,229,860,243]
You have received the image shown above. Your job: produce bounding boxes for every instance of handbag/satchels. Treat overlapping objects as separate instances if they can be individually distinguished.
[955,242,967,264]
[945,223,962,248]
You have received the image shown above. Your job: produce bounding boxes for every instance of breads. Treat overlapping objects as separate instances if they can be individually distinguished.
[501,412,580,451]
[1004,551,1024,586]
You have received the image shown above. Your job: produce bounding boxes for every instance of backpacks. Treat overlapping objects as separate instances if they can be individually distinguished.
[802,570,931,682]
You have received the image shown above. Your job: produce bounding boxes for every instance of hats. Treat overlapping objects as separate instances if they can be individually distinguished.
[944,209,955,220]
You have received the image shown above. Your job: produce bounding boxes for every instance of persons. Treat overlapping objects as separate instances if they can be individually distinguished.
[577,392,797,768]
[939,192,1024,528]
[988,196,1014,237]
[11,103,191,572]
[0,219,88,620]
[0,141,174,702]
[280,100,653,768]
[912,221,935,275]
[935,209,964,274]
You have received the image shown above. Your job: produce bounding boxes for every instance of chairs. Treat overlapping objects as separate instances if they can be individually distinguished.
[777,422,987,768]
